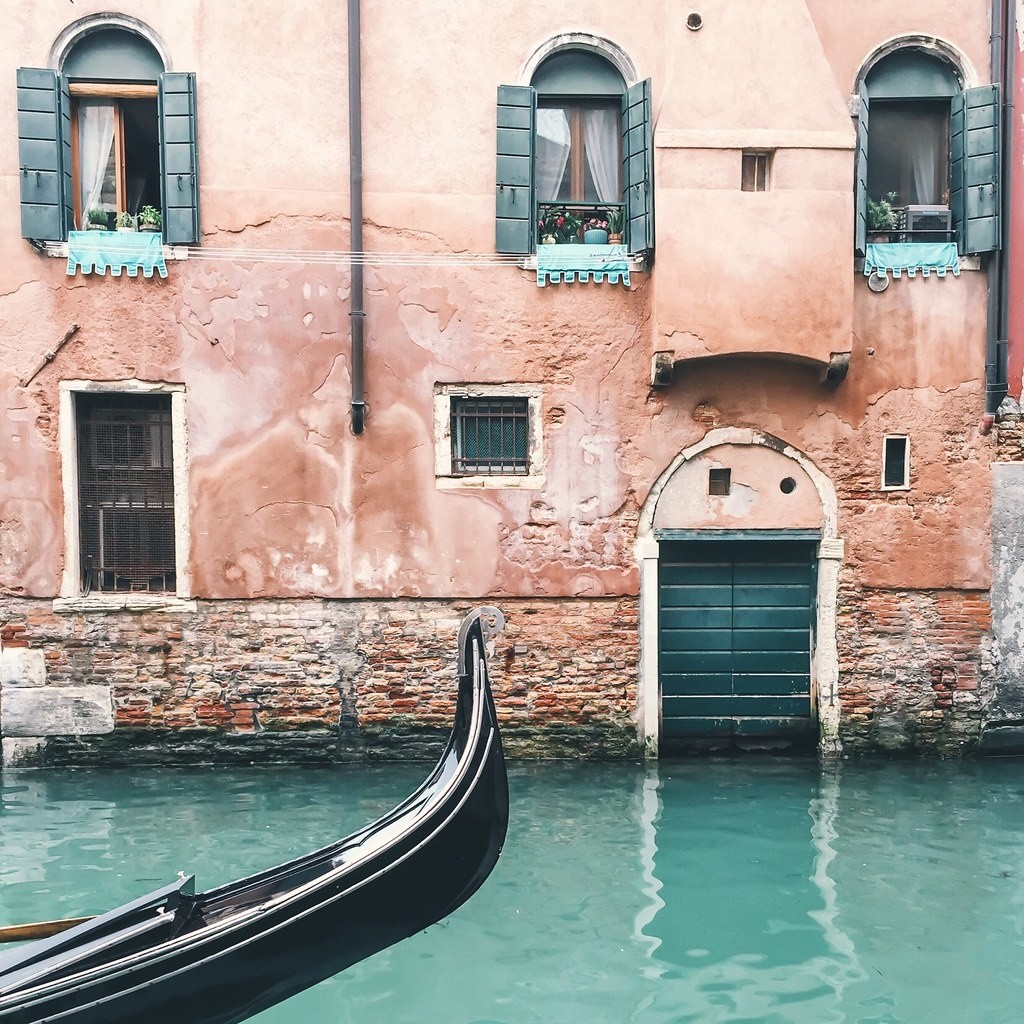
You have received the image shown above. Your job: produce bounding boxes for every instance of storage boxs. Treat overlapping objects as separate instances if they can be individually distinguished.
[899,211,952,243]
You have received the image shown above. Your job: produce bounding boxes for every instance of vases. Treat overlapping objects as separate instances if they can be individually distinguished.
[540,234,556,245]
[583,230,608,244]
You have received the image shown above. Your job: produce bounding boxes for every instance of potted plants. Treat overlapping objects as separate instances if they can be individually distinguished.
[86,208,109,231]
[565,213,582,244]
[608,209,624,245]
[113,205,162,233]
[866,191,905,244]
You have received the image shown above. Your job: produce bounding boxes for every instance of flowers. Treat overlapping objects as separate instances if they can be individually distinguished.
[538,205,564,244]
[583,218,609,231]
